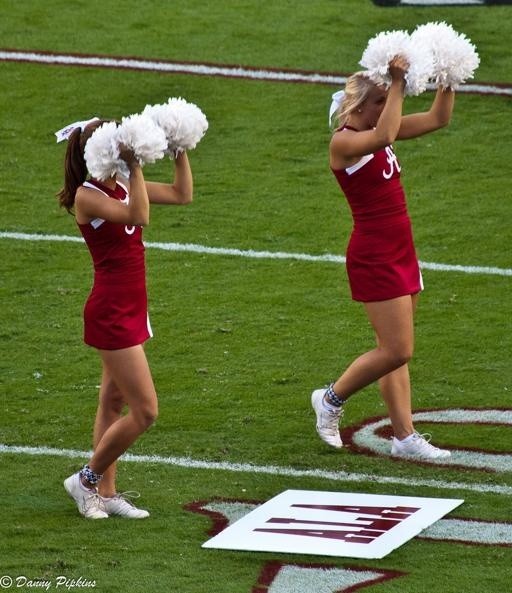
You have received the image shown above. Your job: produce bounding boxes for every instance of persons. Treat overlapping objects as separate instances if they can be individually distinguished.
[56,117,194,522]
[307,52,454,460]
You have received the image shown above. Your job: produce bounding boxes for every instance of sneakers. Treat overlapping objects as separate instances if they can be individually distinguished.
[100,489,152,519]
[63,471,111,520]
[308,387,345,450]
[390,431,454,461]
